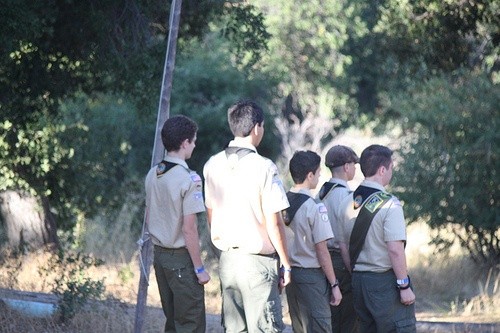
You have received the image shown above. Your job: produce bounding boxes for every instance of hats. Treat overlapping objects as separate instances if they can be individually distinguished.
[326,145,360,167]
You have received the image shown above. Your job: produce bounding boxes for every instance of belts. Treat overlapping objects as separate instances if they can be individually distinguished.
[156,247,188,253]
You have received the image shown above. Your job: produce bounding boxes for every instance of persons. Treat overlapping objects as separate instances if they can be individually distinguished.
[203,97,291,333]
[278,145,418,333]
[145,117,212,333]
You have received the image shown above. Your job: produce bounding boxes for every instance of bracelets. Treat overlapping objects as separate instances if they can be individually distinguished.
[397,278,408,285]
[330,279,339,287]
[398,274,410,290]
[280,267,291,273]
[194,267,205,274]
